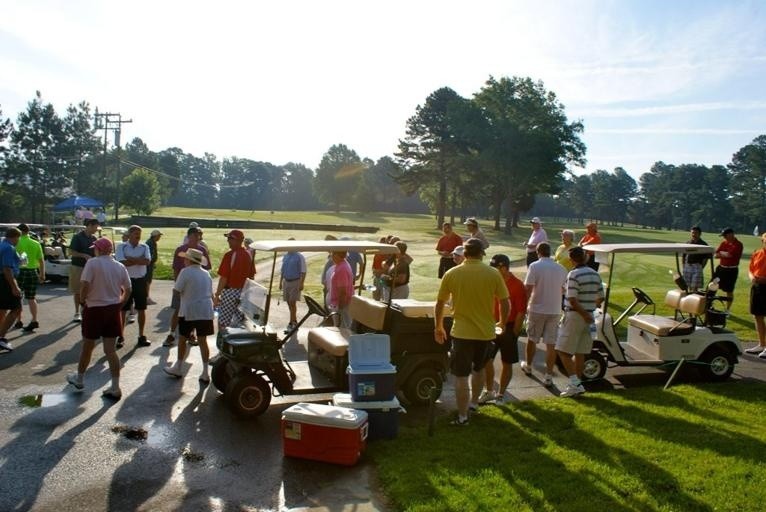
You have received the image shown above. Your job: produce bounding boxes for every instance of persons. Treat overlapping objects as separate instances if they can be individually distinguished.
[1,223,44,353]
[427,218,529,427]
[521,217,605,398]
[683,225,765,359]
[67,236,132,401]
[278,237,307,335]
[163,221,257,380]
[372,236,413,302]
[66,208,165,347]
[322,237,364,334]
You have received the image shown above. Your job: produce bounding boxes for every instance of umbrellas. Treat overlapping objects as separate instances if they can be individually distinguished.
[50,195,107,211]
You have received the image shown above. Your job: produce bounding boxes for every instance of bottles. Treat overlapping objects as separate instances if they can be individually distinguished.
[21,252,27,265]
[708,276,721,291]
[589,312,598,341]
[214,305,220,316]
[668,270,680,281]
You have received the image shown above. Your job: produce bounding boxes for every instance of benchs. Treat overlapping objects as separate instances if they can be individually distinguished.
[626,288,710,339]
[308,293,389,359]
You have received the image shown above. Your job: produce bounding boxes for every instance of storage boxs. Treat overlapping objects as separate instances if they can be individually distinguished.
[366,413,385,442]
[279,398,368,468]
[346,331,402,402]
[332,391,405,440]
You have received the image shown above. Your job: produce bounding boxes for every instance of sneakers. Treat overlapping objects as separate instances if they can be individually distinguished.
[0,337,13,351]
[103,387,121,398]
[451,390,504,425]
[199,374,209,381]
[15,321,38,331]
[163,366,182,377]
[73,311,82,320]
[128,313,135,322]
[68,375,83,389]
[560,384,585,397]
[166,334,199,346]
[521,361,531,375]
[543,378,553,387]
[117,336,152,347]
[745,345,766,358]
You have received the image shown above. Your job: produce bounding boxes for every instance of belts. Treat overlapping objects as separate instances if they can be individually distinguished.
[721,265,737,268]
[569,308,593,312]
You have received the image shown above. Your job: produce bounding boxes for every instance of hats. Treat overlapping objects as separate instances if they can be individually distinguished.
[451,238,509,266]
[178,248,208,266]
[151,230,162,236]
[224,230,244,240]
[719,228,732,236]
[89,238,113,253]
[530,217,540,223]
[464,218,477,225]
